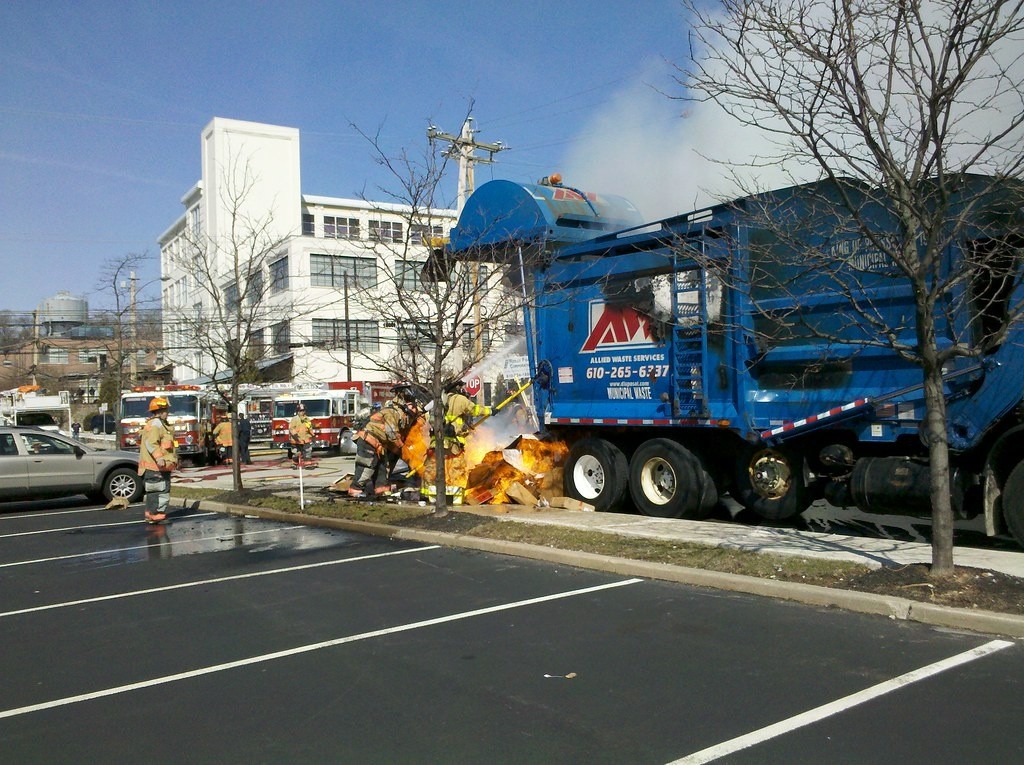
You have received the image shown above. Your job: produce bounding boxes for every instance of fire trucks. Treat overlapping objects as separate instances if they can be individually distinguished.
[270,381,404,459]
[121,382,274,466]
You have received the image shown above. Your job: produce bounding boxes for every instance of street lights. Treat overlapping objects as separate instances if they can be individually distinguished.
[129,274,172,386]
[3,360,37,385]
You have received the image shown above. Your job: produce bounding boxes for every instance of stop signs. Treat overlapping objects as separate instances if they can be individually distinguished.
[460,371,481,398]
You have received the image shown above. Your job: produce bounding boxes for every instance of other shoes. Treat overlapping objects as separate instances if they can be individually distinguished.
[347,492,366,498]
[146,518,172,525]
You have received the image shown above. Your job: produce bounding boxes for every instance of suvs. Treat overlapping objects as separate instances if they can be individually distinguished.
[0,426,147,506]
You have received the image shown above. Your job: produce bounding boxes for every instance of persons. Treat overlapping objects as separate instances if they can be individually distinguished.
[71,419,81,441]
[348,380,426,497]
[138,398,178,520]
[34,444,41,454]
[505,390,529,434]
[212,416,233,465]
[288,403,315,470]
[419,378,500,506]
[238,413,252,464]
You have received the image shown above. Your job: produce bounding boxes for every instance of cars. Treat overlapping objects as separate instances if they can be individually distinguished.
[92,414,119,435]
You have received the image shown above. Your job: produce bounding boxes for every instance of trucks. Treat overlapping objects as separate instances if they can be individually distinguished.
[0,385,74,439]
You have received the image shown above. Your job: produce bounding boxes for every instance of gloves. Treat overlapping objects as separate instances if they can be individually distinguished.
[491,407,500,416]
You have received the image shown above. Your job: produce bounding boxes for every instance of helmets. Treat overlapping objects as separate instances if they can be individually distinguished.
[443,378,466,394]
[389,381,412,393]
[401,401,418,419]
[295,404,307,411]
[146,398,173,412]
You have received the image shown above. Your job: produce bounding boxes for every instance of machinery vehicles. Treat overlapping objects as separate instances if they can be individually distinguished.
[447,172,1024,554]
